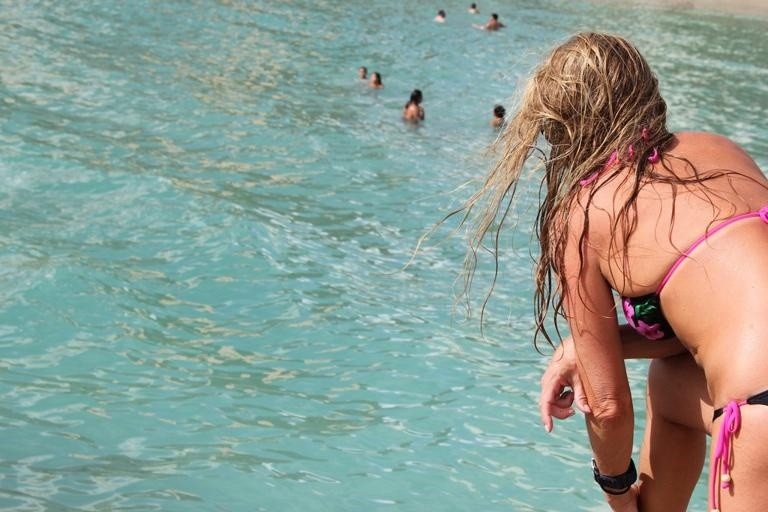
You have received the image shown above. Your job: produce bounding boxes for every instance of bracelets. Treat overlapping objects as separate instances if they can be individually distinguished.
[591,453,638,496]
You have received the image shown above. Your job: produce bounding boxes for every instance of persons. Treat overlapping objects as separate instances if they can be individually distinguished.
[409,35,767,512]
[403,89,424,122]
[489,105,507,127]
[368,73,384,89]
[359,66,367,80]
[471,14,507,31]
[432,9,445,23]
[468,3,479,13]
